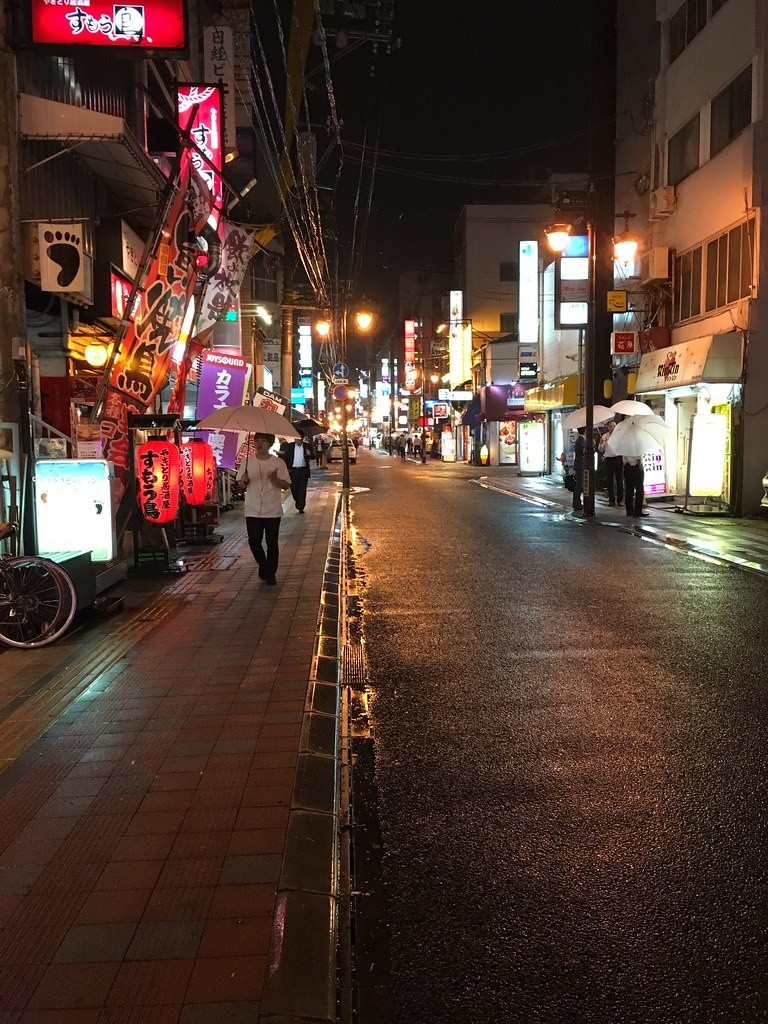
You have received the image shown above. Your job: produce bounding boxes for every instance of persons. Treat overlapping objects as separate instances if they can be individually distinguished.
[572,427,596,510]
[352,437,359,448]
[236,432,292,585]
[315,435,326,466]
[395,434,423,459]
[598,421,649,516]
[272,430,315,513]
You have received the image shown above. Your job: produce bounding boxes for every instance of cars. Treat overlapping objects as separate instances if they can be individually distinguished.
[330,438,357,463]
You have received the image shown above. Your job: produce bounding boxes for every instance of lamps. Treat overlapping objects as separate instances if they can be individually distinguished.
[85,340,107,368]
[107,343,123,364]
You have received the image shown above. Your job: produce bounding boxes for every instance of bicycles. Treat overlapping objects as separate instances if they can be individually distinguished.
[0,522,77,651]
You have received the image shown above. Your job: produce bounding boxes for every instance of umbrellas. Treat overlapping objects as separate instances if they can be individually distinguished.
[565,400,666,456]
[292,419,325,436]
[194,402,301,483]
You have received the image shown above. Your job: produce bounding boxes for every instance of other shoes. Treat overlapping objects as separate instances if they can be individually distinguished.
[574,504,584,510]
[258,560,269,580]
[633,512,650,517]
[608,502,615,506]
[266,570,277,586]
[299,508,305,514]
[295,502,299,510]
[626,511,634,516]
[617,504,623,506]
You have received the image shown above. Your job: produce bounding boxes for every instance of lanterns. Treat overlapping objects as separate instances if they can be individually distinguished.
[180,437,215,505]
[604,380,612,399]
[136,436,180,523]
[627,374,638,394]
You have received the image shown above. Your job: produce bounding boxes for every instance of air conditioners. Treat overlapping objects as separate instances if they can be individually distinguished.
[642,247,669,285]
[649,186,675,219]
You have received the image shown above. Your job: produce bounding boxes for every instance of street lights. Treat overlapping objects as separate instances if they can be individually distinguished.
[436,317,484,466]
[543,181,644,516]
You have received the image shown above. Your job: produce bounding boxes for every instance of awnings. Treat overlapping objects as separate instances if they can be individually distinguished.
[524,375,579,411]
[18,92,175,232]
[634,331,744,394]
[455,392,479,427]
